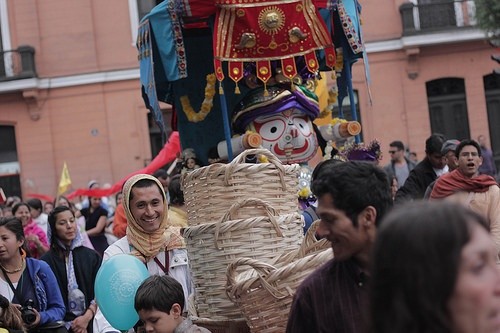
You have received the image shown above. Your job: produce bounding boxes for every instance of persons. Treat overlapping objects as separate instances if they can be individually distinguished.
[133,273,210,333]
[366,198,500,333]
[0,147,211,333]
[283,160,396,333]
[384,132,500,263]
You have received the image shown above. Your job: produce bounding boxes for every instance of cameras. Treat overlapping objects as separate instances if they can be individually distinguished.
[20,298,37,324]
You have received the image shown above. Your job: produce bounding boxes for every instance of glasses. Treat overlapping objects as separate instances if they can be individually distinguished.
[389,151,399,155]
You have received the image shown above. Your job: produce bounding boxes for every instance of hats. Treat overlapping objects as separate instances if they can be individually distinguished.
[441,140,461,156]
[426,133,449,153]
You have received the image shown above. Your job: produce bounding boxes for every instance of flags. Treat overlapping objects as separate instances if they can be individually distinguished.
[58,162,72,196]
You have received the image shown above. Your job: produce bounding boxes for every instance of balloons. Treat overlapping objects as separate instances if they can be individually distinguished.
[93,252,151,332]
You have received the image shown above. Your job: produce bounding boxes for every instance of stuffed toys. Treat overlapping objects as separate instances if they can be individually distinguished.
[232,84,319,168]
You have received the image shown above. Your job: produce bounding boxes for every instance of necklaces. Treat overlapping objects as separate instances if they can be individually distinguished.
[1,259,24,274]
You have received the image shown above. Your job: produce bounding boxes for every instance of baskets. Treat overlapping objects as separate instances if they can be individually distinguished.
[180,149,335,333]
